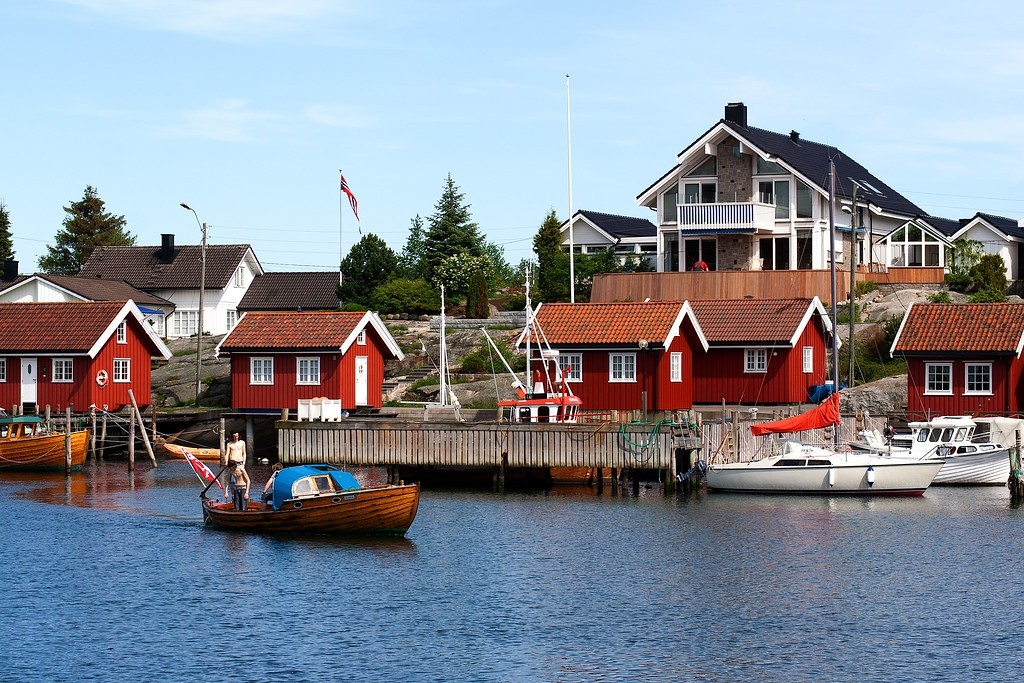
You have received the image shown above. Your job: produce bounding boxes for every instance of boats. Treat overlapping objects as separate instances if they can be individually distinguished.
[844,408,1017,485]
[163,443,220,460]
[0,417,89,471]
[481,267,584,424]
[201,462,421,543]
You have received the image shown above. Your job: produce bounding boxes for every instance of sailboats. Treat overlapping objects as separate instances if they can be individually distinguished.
[708,158,949,498]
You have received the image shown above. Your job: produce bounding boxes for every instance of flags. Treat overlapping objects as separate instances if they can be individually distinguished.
[181,447,224,490]
[340,173,364,235]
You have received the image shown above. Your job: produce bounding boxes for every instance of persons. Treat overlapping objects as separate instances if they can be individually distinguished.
[261,461,285,501]
[223,430,247,501]
[225,458,251,512]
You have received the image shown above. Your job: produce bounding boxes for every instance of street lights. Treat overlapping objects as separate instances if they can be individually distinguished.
[839,182,867,388]
[179,203,206,405]
